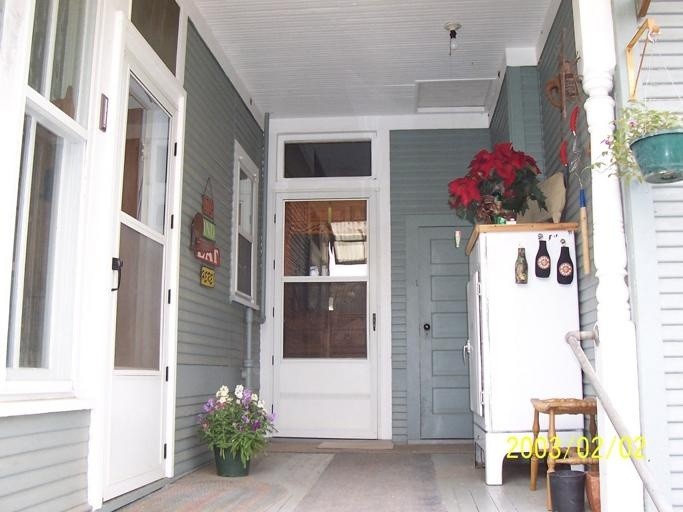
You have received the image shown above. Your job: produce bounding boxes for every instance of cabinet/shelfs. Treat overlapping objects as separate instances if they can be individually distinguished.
[463,220,587,484]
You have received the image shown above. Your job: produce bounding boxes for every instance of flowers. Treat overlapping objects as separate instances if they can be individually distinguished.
[444,143,547,224]
[196,385,277,468]
[595,105,683,184]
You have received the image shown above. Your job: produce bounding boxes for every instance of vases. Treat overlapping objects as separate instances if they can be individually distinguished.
[628,127,682,184]
[548,469,601,512]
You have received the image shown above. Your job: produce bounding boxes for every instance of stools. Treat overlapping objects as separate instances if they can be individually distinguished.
[528,395,597,511]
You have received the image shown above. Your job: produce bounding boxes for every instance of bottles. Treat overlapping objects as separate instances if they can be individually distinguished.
[515,247,529,285]
[556,246,574,285]
[534,240,551,278]
